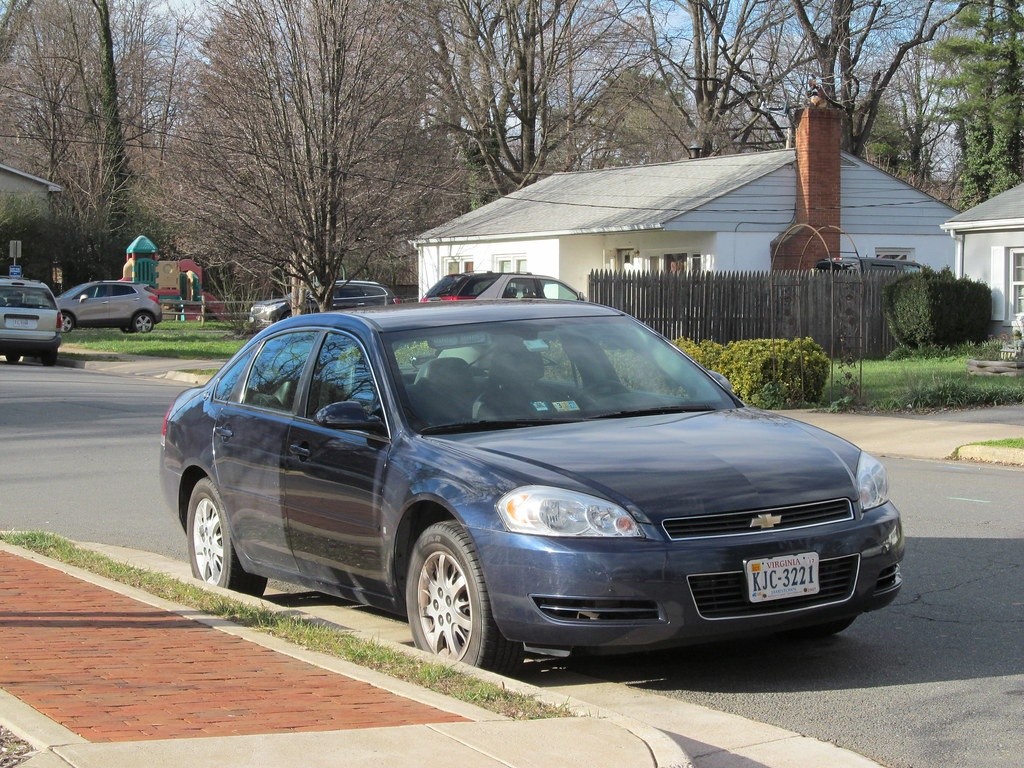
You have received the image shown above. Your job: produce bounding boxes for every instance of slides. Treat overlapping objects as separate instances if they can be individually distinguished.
[202,291,240,321]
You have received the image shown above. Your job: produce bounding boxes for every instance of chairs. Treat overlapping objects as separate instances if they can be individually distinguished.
[404,340,603,427]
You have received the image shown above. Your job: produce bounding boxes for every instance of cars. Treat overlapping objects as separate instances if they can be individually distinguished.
[159,298,905,679]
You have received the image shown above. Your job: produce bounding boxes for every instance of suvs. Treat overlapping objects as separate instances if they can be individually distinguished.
[248,280,401,333]
[0,275,64,366]
[53,280,163,335]
[420,270,589,355]
